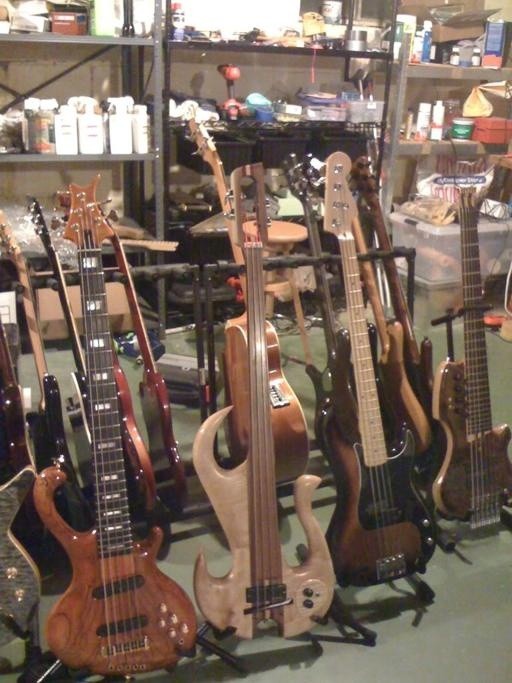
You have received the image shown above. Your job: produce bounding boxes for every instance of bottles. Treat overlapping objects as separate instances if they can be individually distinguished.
[423,21,432,64]
[403,107,415,140]
[171,2,185,41]
[450,46,461,66]
[472,47,481,68]
[416,103,431,143]
[432,102,445,142]
[132,104,152,153]
[20,96,59,155]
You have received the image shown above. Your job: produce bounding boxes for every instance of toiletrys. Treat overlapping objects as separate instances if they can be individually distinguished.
[131,103,147,154]
[106,96,134,155]
[78,98,103,156]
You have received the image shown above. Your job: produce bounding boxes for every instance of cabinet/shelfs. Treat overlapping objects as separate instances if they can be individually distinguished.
[382,32,512,222]
[0,1,165,340]
[164,0,398,329]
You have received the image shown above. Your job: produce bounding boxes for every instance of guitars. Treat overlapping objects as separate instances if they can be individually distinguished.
[0,211,95,531]
[190,120,309,487]
[111,343,157,521]
[95,197,186,512]
[33,174,195,676]
[352,214,431,458]
[432,186,512,529]
[314,149,436,588]
[350,157,432,453]
[28,194,96,529]
[281,152,378,465]
[193,167,336,634]
[0,318,49,550]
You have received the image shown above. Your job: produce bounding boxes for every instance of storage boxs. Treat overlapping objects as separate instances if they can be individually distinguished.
[388,210,512,282]
[471,115,512,146]
[398,0,503,43]
[36,281,135,342]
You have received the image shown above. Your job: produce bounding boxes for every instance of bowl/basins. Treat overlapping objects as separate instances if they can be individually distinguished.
[451,119,473,142]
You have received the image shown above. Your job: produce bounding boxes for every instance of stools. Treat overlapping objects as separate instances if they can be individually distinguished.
[241,218,315,366]
